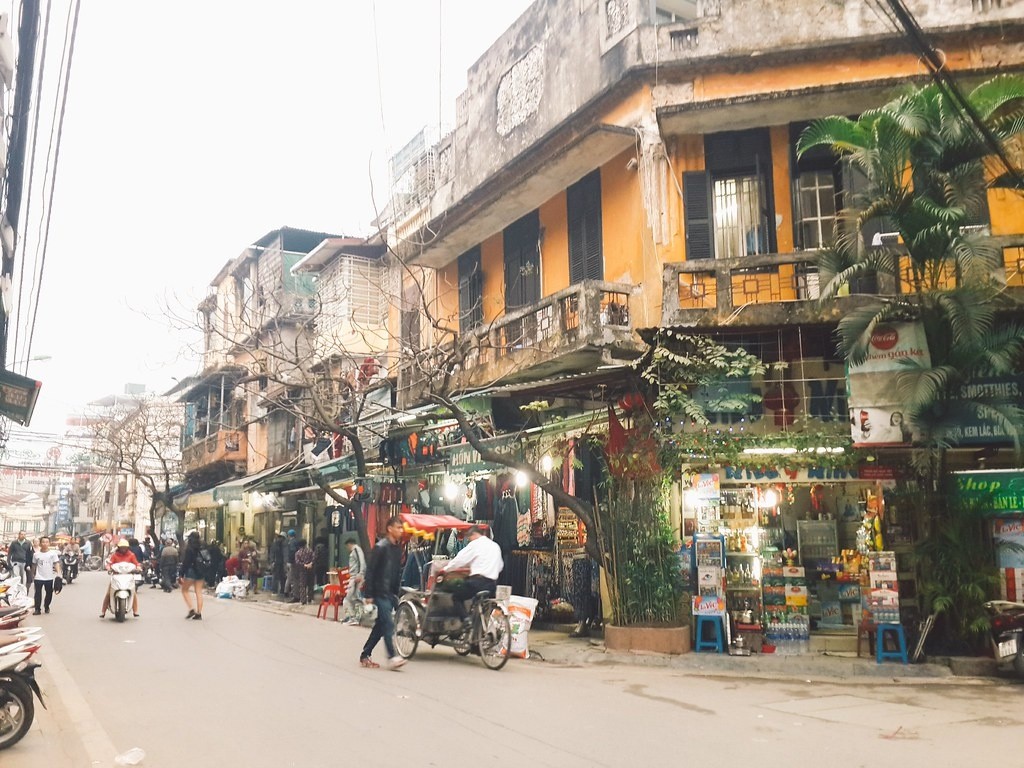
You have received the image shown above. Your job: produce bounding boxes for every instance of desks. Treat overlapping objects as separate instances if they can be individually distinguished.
[326,571,348,585]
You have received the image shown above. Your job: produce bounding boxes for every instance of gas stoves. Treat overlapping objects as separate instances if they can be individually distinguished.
[729,646,751,656]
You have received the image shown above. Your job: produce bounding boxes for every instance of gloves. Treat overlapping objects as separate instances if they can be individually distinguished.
[435,570,448,583]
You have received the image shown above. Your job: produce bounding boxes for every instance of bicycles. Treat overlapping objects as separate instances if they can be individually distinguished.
[80,554,102,571]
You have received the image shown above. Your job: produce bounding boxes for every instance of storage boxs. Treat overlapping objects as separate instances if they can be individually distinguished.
[785,586,812,606]
[869,571,898,592]
[762,568,784,586]
[691,594,727,616]
[873,604,901,624]
[860,587,873,620]
[869,551,897,571]
[784,577,808,586]
[698,586,723,595]
[822,599,844,625]
[871,587,901,605]
[787,606,807,615]
[851,604,861,626]
[783,565,806,577]
[763,586,785,606]
[697,565,720,585]
[839,582,861,603]
[788,614,810,624]
[764,604,786,624]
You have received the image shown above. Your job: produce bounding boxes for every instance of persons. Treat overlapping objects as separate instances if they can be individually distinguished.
[63,537,79,578]
[273,531,287,594]
[7,530,32,586]
[239,540,257,594]
[159,539,166,552]
[437,527,504,630]
[128,539,143,563]
[360,518,408,670]
[295,539,314,605]
[160,538,178,592]
[178,530,205,619]
[341,538,366,625]
[80,537,92,562]
[284,530,299,598]
[206,542,221,587]
[143,537,151,560]
[31,536,61,615]
[98,539,139,617]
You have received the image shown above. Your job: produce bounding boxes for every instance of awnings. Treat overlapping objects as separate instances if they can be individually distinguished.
[0,368,42,426]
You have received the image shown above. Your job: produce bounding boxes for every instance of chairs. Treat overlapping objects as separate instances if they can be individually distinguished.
[316,584,344,622]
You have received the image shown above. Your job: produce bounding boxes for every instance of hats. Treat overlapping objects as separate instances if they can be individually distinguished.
[186,531,200,539]
[277,531,287,539]
[287,529,296,536]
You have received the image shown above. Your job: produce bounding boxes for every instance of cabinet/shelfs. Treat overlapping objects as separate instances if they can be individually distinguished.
[795,518,840,570]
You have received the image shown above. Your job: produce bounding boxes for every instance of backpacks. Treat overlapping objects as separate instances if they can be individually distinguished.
[196,549,212,570]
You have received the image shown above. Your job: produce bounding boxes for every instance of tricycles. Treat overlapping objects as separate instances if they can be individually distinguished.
[393,511,511,670]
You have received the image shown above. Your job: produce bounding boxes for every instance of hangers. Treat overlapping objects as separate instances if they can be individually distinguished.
[502,489,512,499]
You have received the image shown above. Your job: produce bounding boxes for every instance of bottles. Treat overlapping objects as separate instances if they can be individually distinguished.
[700,496,785,609]
[801,534,900,621]
[764,612,809,653]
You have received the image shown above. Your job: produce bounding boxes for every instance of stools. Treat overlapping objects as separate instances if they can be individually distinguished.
[875,621,911,665]
[694,613,727,654]
[261,575,273,592]
[857,621,900,659]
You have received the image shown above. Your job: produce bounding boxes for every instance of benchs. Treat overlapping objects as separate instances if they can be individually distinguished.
[721,487,764,633]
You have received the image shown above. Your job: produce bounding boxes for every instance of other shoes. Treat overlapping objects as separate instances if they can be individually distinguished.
[343,617,359,626]
[45,607,50,614]
[457,620,474,634]
[133,612,139,617]
[99,614,106,618]
[388,656,407,670]
[359,656,379,668]
[33,609,41,615]
[186,610,195,618]
[192,614,202,620]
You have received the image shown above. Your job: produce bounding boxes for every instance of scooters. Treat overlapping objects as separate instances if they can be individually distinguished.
[982,599,1024,681]
[0,553,48,751]
[105,560,143,623]
[57,551,79,584]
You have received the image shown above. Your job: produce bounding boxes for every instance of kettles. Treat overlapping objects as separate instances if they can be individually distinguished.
[732,634,746,647]
[737,603,754,624]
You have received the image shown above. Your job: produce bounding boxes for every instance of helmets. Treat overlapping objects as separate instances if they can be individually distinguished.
[117,539,129,548]
